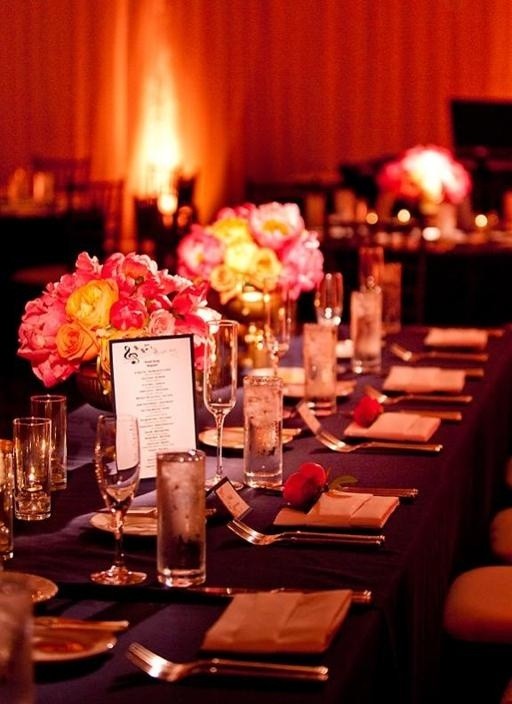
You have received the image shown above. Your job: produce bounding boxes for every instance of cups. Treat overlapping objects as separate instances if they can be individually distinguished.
[12,416,53,522]
[357,246,384,289]
[0,581,38,703]
[313,271,347,376]
[371,262,402,335]
[243,376,285,488]
[301,322,338,417]
[155,448,207,587]
[350,290,383,375]
[30,393,69,492]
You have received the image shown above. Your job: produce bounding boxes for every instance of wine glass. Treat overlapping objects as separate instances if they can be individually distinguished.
[90,414,148,587]
[260,276,292,421]
[202,318,244,493]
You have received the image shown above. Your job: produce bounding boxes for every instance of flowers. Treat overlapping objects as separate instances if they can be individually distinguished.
[176,201,324,305]
[375,143,473,215]
[16,252,223,395]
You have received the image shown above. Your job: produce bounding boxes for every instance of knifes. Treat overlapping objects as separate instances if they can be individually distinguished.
[188,585,372,604]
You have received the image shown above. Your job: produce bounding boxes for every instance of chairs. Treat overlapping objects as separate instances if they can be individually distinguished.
[13,154,93,203]
[62,179,124,264]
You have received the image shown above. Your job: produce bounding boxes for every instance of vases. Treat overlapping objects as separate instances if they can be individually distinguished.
[223,286,281,368]
[75,359,113,402]
[427,209,455,229]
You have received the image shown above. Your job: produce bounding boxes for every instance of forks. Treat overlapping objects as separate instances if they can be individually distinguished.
[314,428,444,453]
[125,641,329,682]
[225,516,386,546]
[363,383,473,403]
[390,342,489,362]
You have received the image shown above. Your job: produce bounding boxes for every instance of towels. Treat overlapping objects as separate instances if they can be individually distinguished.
[200,585,354,652]
[424,327,489,350]
[381,366,465,392]
[343,411,439,443]
[273,485,400,530]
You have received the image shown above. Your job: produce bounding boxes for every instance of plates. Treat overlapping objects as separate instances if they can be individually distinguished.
[33,615,116,662]
[0,569,59,603]
[198,426,295,450]
[89,505,156,536]
[0,439,19,561]
[250,365,306,383]
[336,339,352,358]
[283,381,354,398]
